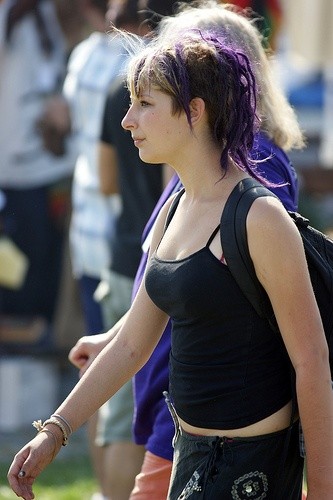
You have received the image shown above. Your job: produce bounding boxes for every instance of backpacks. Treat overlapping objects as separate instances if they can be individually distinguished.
[219,177,333,381]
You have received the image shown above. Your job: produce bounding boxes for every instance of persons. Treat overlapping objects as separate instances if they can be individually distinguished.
[68,0,308,499]
[4,25,332,500]
[0,1,181,500]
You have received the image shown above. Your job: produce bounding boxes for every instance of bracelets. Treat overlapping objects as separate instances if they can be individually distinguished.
[32,413,73,453]
